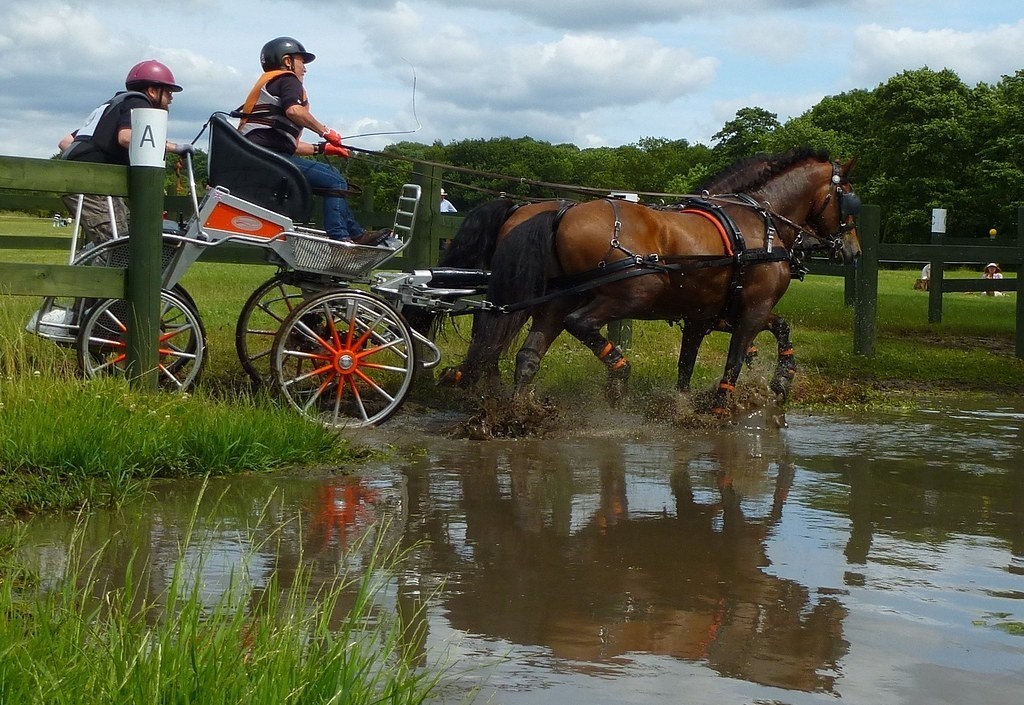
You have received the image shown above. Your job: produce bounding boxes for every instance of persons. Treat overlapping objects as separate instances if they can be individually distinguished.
[980,262,1009,297]
[440,189,458,213]
[58,60,195,245]
[235,36,394,256]
[919,263,947,282]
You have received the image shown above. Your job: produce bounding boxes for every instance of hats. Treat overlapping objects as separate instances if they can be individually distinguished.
[985,263,1001,273]
[441,188,448,196]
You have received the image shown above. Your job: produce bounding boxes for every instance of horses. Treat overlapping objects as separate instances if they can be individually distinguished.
[397,147,865,430]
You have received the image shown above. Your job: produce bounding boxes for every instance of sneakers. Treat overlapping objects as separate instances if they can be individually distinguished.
[355,228,391,246]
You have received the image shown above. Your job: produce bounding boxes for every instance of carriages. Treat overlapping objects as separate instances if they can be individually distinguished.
[24,143,865,432]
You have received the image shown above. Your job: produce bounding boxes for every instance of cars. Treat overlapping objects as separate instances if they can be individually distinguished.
[162,219,186,245]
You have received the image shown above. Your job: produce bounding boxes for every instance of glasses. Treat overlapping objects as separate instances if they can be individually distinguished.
[156,85,174,96]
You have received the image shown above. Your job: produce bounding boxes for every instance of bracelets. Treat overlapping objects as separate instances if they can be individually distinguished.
[311,143,319,156]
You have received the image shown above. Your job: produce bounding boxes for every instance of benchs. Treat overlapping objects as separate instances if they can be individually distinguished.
[208,112,315,224]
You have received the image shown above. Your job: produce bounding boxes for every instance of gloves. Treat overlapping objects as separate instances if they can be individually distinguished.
[177,142,194,158]
[319,125,342,147]
[324,142,350,158]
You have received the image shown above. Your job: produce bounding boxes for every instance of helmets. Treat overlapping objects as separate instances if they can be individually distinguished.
[126,60,183,92]
[260,36,315,73]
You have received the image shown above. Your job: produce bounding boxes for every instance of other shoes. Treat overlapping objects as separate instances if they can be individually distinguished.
[69,321,79,336]
